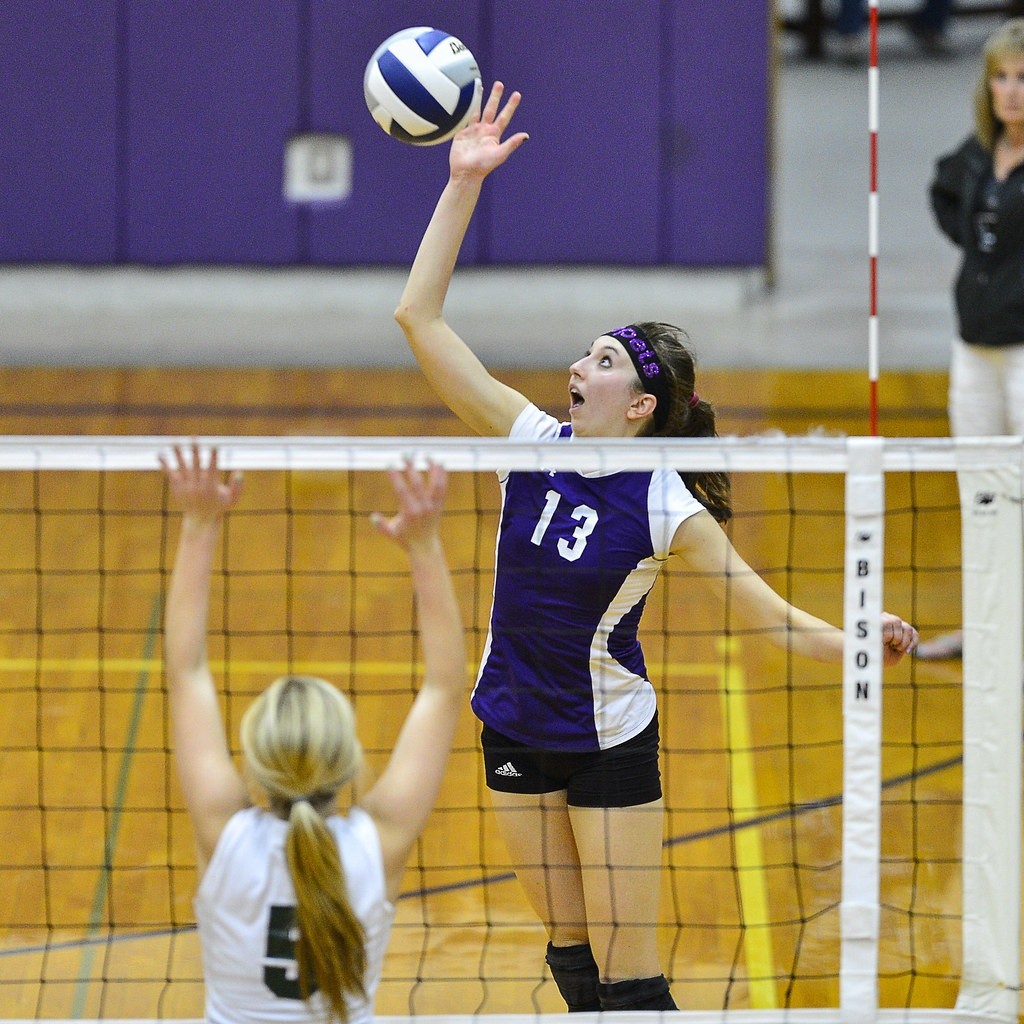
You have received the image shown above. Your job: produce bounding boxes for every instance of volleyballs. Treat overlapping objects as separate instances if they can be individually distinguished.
[362,26,483,147]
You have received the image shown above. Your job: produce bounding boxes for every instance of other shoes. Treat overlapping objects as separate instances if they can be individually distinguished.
[912,628,962,659]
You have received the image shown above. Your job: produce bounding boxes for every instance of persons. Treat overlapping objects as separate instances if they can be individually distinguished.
[392,75,921,1012]
[157,441,465,1021]
[914,20,1022,660]
